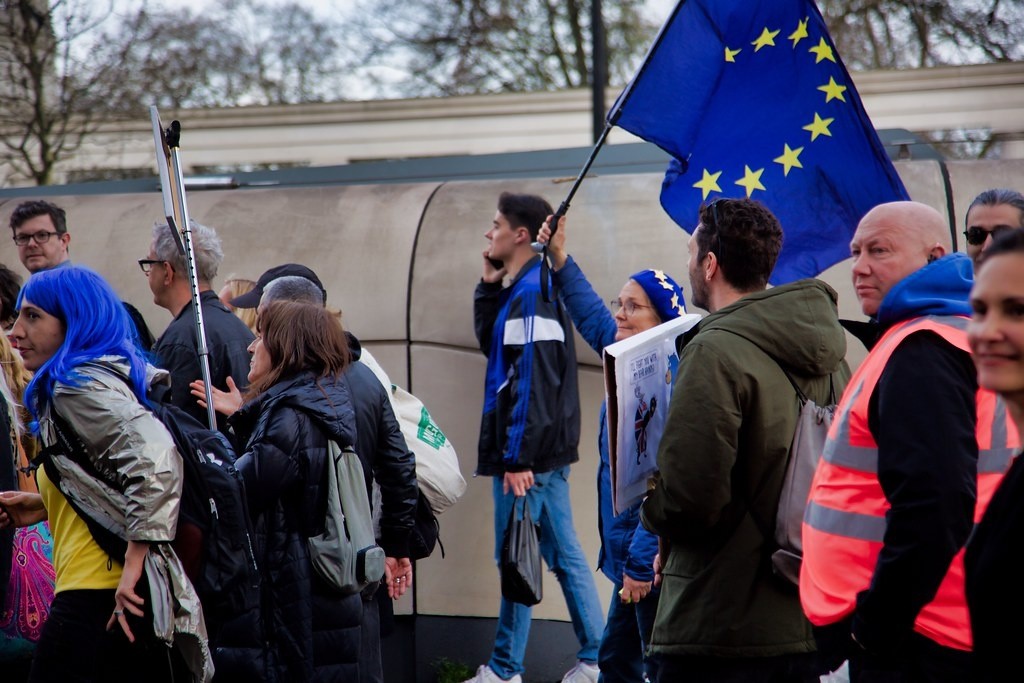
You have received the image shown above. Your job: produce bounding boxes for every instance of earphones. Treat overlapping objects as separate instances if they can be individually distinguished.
[929,254,938,264]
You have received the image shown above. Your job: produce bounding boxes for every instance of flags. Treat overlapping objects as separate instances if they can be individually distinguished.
[605,0,910,286]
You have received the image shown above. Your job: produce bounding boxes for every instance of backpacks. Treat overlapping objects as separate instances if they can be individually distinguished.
[50,359,259,600]
[308,433,386,600]
[771,363,839,589]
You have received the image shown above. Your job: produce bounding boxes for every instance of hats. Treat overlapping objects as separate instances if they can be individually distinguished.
[630,269,686,323]
[229,263,327,308]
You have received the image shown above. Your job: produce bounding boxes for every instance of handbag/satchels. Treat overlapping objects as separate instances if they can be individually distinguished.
[501,497,543,606]
[392,383,466,514]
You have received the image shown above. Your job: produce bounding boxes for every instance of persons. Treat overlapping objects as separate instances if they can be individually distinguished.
[640,196,852,683]
[0,200,392,683]
[189,301,419,683]
[798,189,1024,683]
[598,268,687,683]
[0,266,214,683]
[463,192,605,683]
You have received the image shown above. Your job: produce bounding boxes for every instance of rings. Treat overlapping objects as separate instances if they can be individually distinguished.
[395,576,405,583]
[113,611,123,616]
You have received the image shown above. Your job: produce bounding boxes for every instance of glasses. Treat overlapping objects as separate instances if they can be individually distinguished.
[138,259,176,273]
[13,230,65,245]
[611,301,654,315]
[963,228,1012,245]
[706,198,729,263]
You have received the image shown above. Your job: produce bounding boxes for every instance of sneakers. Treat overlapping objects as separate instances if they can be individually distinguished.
[561,661,599,683]
[464,664,522,683]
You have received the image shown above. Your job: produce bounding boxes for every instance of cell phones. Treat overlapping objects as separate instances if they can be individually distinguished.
[486,256,503,271]
